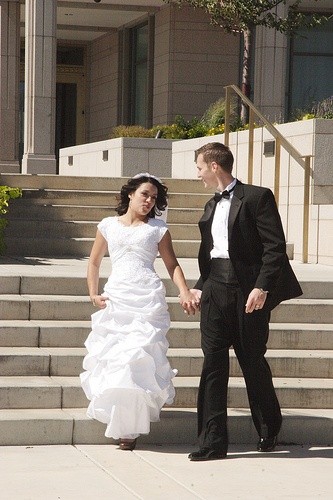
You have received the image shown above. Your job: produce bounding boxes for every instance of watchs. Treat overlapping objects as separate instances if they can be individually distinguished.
[260,288,269,294]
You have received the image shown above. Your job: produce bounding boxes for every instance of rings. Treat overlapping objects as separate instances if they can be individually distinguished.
[256,306,259,310]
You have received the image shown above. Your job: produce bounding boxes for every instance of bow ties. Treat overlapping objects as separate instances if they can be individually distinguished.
[213,185,235,202]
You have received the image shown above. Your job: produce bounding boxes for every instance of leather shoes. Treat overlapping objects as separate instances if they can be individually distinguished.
[258,436,277,452]
[188,447,227,460]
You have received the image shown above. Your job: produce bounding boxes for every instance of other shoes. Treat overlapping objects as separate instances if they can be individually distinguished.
[119,439,136,450]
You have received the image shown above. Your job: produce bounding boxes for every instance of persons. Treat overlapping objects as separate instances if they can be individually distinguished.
[179,142,302,460]
[79,171,196,450]
